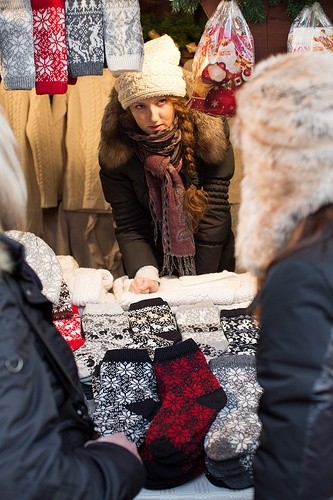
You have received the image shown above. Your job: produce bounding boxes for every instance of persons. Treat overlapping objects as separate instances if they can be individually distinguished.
[237,49,332,500]
[0,106,148,500]
[97,35,237,294]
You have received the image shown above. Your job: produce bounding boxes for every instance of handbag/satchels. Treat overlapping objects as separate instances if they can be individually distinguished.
[287,2,333,54]
[187,1,255,119]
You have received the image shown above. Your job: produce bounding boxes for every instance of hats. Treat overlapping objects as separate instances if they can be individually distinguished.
[111,34,187,110]
[229,52,333,276]
[4,228,63,306]
